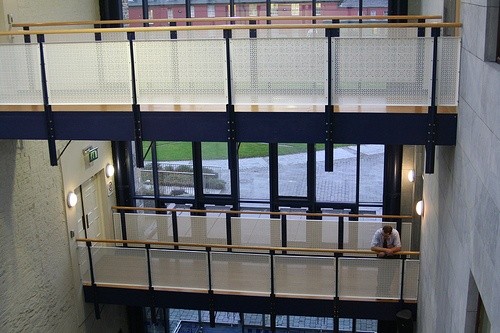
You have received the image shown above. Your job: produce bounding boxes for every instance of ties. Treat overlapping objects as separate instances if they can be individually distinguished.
[383,240,388,250]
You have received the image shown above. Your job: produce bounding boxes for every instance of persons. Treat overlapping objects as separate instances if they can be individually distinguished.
[369,224,401,300]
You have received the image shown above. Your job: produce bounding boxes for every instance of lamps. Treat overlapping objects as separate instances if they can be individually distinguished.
[415,200,424,217]
[67,191,77,208]
[408,169,415,183]
[106,163,114,178]
